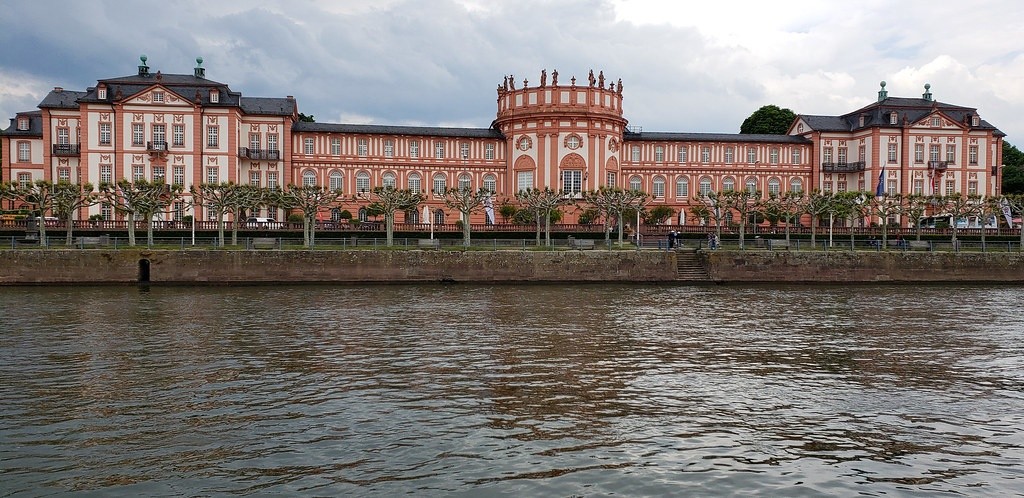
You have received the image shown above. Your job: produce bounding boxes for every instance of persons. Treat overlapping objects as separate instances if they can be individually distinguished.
[240,217,247,230]
[668,231,675,248]
[330,218,335,228]
[540,69,558,86]
[617,78,623,91]
[712,231,719,247]
[588,69,604,87]
[504,75,515,91]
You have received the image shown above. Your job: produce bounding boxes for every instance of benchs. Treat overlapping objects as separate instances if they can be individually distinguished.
[74,237,100,249]
[767,239,790,251]
[418,239,441,251]
[908,240,931,251]
[252,238,275,250]
[14,238,39,248]
[571,239,596,250]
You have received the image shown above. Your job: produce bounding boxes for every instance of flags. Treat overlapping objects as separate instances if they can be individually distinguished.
[930,177,934,196]
[876,167,884,196]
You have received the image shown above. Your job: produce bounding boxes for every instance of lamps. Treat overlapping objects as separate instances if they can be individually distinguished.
[560,171,562,180]
[583,172,588,180]
